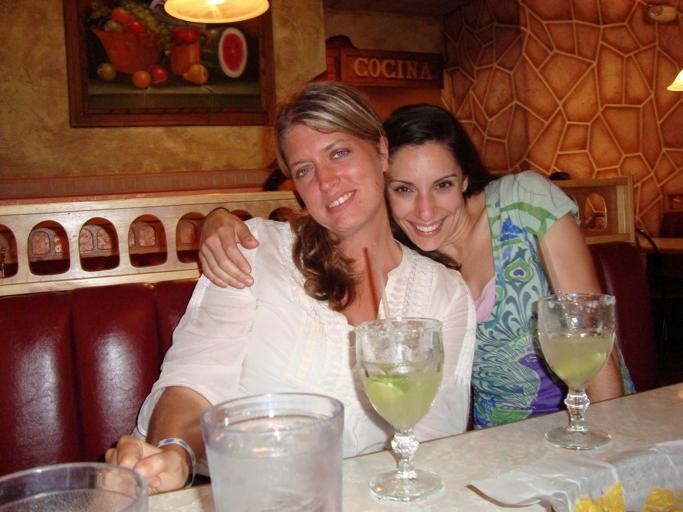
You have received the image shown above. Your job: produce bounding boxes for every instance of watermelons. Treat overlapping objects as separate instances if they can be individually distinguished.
[217,26,248,79]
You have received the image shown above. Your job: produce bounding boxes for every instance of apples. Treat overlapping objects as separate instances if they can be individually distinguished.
[146,64,169,85]
[127,21,145,32]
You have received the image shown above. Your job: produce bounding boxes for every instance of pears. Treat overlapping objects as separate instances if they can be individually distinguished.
[182,64,208,85]
[104,21,122,32]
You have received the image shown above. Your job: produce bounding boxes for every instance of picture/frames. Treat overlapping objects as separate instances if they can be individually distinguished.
[61,0,277,127]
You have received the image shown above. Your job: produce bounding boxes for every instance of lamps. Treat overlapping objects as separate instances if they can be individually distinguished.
[665,67,683,92]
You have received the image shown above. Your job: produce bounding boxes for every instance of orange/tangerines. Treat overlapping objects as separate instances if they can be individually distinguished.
[97,62,116,80]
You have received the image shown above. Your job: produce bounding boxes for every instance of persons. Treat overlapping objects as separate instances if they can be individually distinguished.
[198,103,638,430]
[94,84,475,499]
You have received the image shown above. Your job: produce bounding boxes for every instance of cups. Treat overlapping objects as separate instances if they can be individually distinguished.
[202,391,345,512]
[0,461,149,512]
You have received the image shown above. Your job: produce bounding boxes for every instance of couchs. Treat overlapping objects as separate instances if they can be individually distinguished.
[0,241,655,500]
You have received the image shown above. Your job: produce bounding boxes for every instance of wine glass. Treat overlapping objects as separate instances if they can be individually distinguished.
[537,292,616,451]
[356,316,445,503]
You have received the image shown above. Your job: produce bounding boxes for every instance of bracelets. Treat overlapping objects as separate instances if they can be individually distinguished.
[153,437,196,488]
